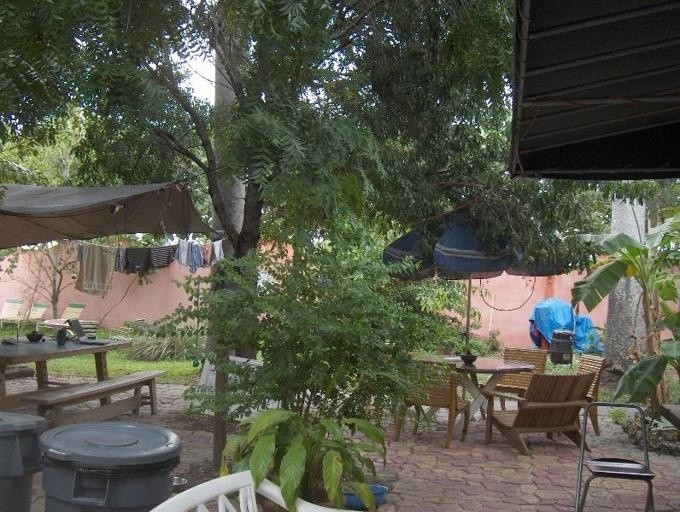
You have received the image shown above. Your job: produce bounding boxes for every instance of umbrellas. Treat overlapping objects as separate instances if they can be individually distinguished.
[383,193,578,400]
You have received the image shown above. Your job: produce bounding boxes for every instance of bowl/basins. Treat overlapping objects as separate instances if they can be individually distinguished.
[460,355,477,365]
[25,333,44,342]
[342,483,390,509]
[171,476,187,492]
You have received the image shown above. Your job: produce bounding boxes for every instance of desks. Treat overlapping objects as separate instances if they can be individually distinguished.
[0,337,132,417]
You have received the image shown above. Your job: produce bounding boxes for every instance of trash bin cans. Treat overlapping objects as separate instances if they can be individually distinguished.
[551,331,574,369]
[0,411,182,512]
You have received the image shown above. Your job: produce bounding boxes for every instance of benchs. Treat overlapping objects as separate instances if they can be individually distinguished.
[22,372,166,427]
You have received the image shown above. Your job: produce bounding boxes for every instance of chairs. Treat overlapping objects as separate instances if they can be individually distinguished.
[391,347,604,456]
[1,299,87,338]
[574,402,656,511]
